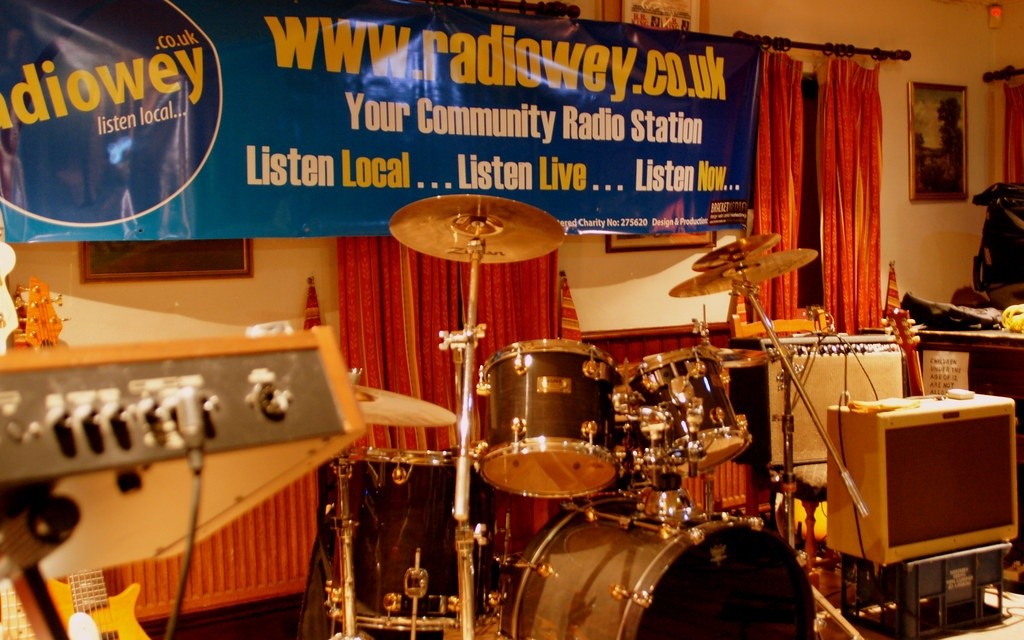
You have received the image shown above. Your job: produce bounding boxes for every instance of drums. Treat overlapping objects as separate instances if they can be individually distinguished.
[500,493,819,640]
[469,337,624,499]
[323,443,496,631]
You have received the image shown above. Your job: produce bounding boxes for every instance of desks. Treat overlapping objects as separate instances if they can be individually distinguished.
[857,326,1024,564]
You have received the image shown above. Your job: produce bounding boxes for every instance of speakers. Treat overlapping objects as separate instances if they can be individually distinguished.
[726,334,908,467]
[828,392,1018,564]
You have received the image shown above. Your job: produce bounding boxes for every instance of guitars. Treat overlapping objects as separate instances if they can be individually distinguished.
[624,345,755,491]
[879,306,928,397]
[9,276,151,639]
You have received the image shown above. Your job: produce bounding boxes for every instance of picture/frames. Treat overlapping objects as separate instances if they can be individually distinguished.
[907,81,969,202]
[78,238,255,285]
[594,0,710,34]
[605,230,718,253]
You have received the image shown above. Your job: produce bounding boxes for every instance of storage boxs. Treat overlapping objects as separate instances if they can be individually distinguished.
[841,542,1013,640]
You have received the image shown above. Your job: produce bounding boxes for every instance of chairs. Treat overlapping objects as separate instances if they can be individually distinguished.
[730,308,827,572]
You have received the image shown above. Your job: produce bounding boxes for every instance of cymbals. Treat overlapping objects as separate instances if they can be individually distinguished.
[668,246,818,299]
[388,192,565,263]
[691,232,782,273]
[351,383,458,427]
[642,347,773,369]
